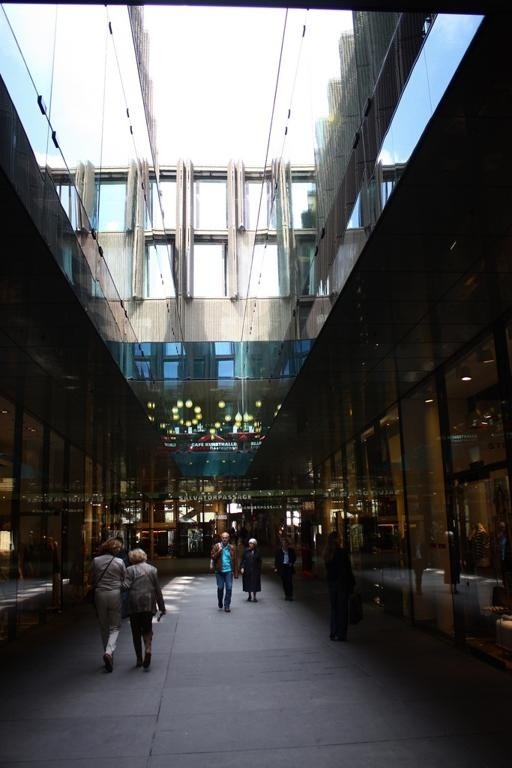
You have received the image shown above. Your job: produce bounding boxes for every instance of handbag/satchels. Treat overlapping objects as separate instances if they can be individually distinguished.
[209,558,215,574]
[84,588,96,603]
[350,596,363,625]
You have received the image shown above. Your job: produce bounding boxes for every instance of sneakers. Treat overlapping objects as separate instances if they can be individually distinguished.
[248,596,257,601]
[218,603,231,612]
[137,652,151,668]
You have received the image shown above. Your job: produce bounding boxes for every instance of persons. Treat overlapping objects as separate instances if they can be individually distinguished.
[441,531,461,594]
[238,538,262,602]
[496,521,510,577]
[272,540,296,601]
[321,532,357,642]
[121,547,167,668]
[86,539,127,673]
[210,532,237,612]
[51,540,62,605]
[411,531,428,595]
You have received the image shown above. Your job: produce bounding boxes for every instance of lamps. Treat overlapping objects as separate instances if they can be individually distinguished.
[461,369,472,381]
[424,393,433,403]
[480,347,495,364]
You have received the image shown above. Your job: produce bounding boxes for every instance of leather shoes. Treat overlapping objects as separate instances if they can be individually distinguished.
[103,654,113,673]
